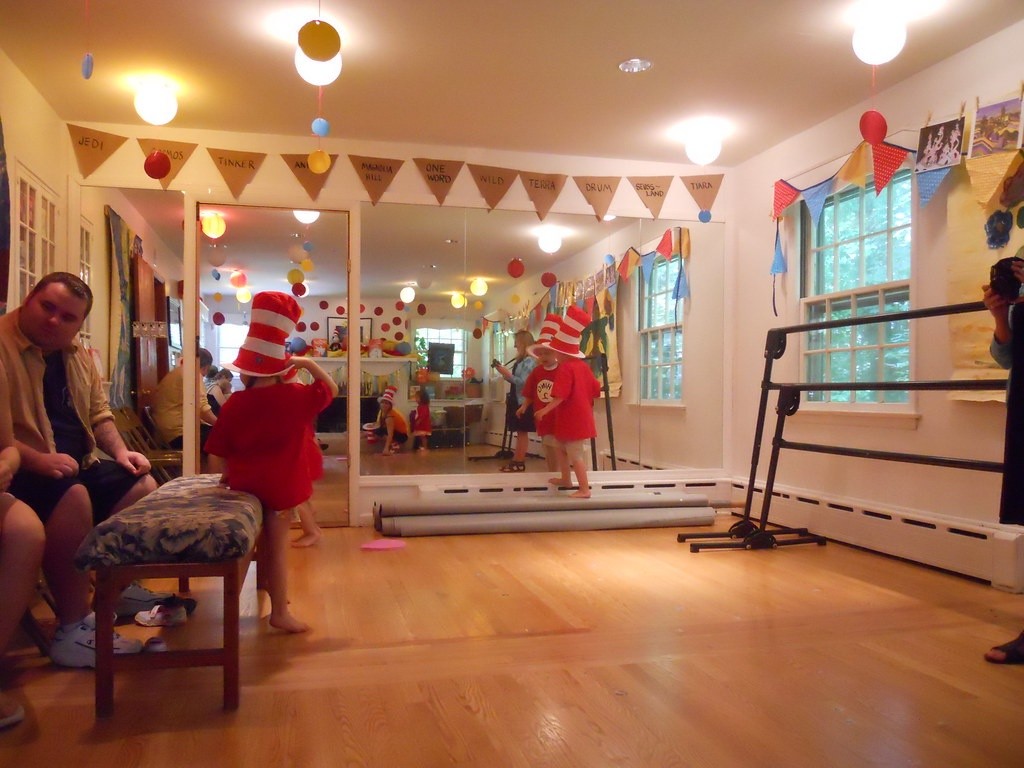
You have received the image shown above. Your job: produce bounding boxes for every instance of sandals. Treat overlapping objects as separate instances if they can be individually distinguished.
[984,632,1024,664]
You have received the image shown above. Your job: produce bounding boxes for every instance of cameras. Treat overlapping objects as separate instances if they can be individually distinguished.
[491,359,502,368]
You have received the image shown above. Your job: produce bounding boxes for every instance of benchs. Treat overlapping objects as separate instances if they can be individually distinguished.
[76,473,260,720]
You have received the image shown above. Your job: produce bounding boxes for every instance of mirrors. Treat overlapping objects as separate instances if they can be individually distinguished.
[196,202,351,528]
[359,200,725,477]
[73,181,185,493]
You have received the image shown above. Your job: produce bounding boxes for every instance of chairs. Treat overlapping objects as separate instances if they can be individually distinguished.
[111,407,184,484]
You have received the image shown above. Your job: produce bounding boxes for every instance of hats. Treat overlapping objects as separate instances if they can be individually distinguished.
[219,291,302,376]
[541,304,591,359]
[362,422,380,443]
[377,386,397,404]
[526,315,561,359]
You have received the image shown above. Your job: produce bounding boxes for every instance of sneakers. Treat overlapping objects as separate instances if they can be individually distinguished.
[90,584,175,611]
[48,613,143,667]
[500,461,525,472]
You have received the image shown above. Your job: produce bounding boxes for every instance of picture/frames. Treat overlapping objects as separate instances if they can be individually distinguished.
[326,318,372,349]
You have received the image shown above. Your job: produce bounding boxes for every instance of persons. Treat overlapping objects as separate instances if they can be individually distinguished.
[203,290,339,632]
[150,350,218,450]
[534,305,601,498]
[411,390,432,453]
[500,331,542,473]
[278,337,321,547]
[372,385,408,456]
[205,349,234,475]
[984,244,1024,665]
[1,271,196,729]
[515,312,563,472]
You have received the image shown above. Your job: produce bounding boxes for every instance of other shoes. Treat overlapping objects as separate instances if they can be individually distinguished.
[0,693,26,730]
[135,604,186,626]
[391,445,399,453]
